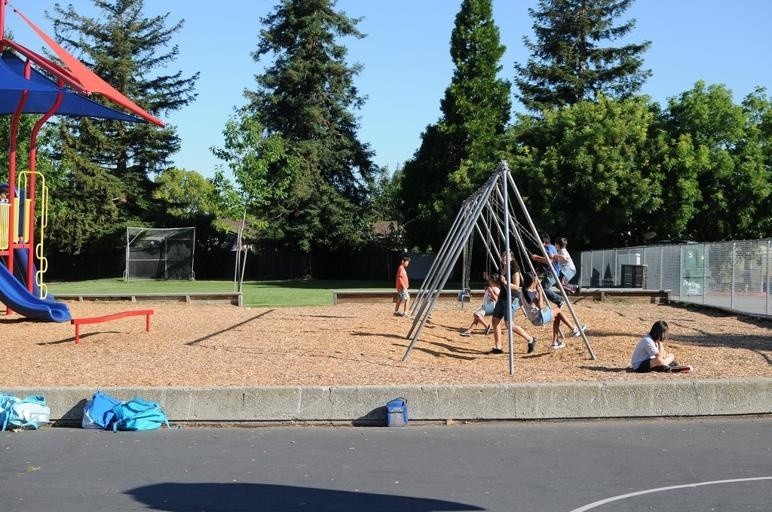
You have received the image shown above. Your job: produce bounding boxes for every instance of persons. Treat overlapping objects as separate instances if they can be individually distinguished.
[392,256,412,316]
[630,321,690,373]
[460,231,587,354]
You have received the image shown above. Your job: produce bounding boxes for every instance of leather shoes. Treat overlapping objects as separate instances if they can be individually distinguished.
[527,337,537,353]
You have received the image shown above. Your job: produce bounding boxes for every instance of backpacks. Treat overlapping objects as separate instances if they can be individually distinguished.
[81,389,174,433]
[0,391,51,432]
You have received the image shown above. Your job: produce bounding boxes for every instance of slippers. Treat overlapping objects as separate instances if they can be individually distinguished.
[486,348,503,354]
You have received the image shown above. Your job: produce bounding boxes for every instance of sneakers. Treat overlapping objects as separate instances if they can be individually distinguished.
[558,299,566,310]
[670,364,691,372]
[459,325,491,337]
[551,342,565,349]
[571,324,586,337]
[393,312,410,318]
